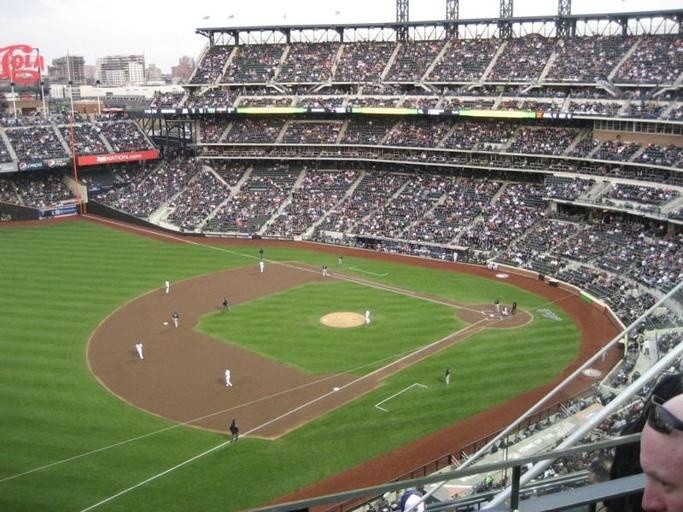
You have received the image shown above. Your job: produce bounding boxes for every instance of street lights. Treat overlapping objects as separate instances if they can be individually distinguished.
[39,79,47,123]
[67,78,75,120]
[94,79,102,118]
[8,79,18,124]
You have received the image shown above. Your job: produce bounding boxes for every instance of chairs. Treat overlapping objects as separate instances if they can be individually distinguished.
[159,39,683,271]
[0,91,161,228]
[535,271,682,343]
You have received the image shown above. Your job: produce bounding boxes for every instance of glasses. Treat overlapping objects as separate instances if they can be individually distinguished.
[642,394,683,435]
